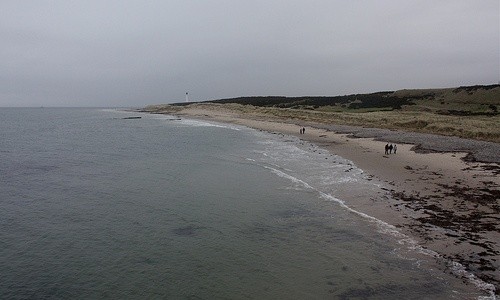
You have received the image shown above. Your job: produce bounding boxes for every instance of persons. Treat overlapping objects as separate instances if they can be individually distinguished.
[384,143,389,155]
[393,144,397,154]
[388,144,393,154]
[302,126,305,134]
